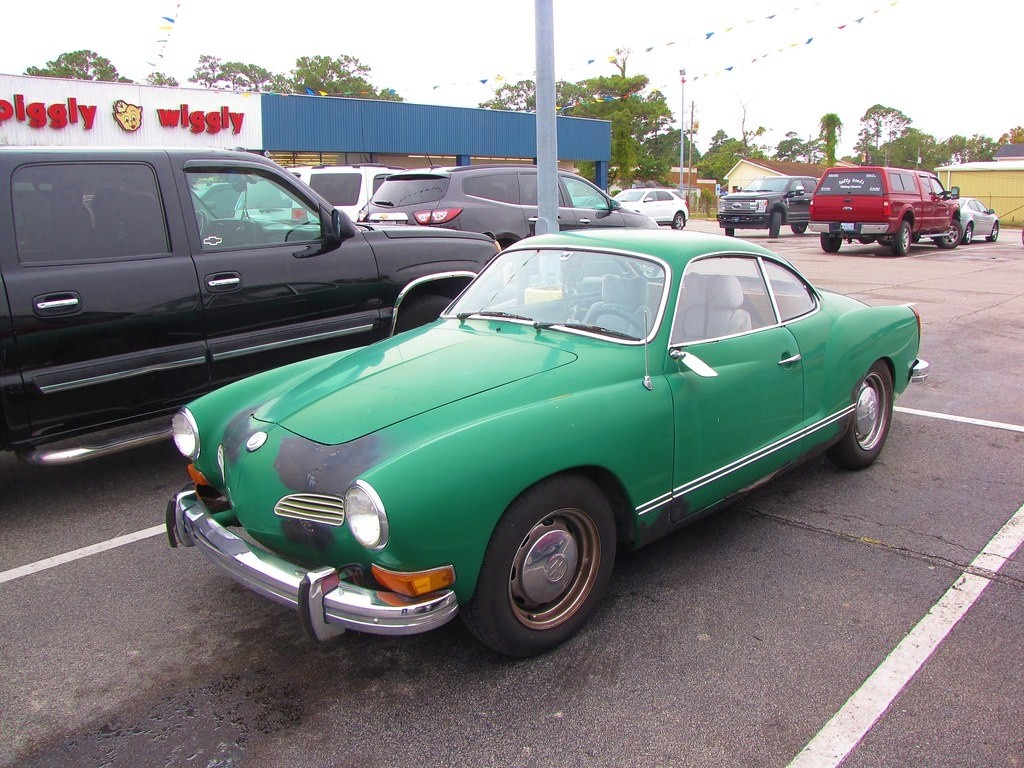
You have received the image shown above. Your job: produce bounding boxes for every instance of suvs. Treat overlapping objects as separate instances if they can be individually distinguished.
[233,163,405,225]
[808,167,963,256]
[717,175,817,238]
[358,164,661,251]
[0,146,503,468]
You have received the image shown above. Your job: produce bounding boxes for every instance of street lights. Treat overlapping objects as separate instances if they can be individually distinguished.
[679,69,685,196]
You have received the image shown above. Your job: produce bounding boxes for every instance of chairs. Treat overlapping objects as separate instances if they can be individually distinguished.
[581,273,652,339]
[671,272,753,344]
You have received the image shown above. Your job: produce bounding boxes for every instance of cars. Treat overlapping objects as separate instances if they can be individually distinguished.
[613,188,689,231]
[192,182,240,220]
[956,198,1000,244]
[163,230,931,658]
